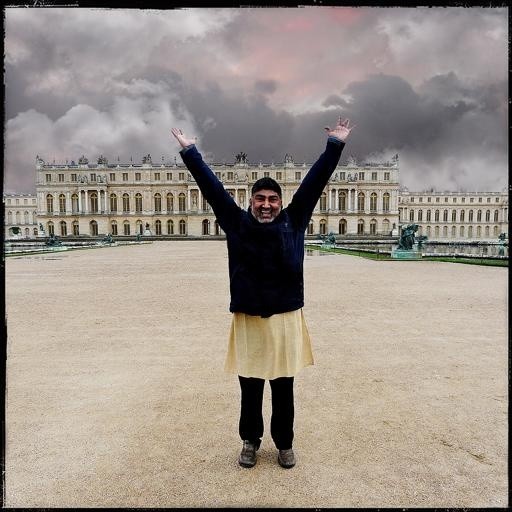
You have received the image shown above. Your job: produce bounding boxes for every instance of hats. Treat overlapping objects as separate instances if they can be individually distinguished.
[252,177,282,199]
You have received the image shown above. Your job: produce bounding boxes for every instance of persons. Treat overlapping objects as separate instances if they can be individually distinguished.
[170,115,357,469]
[396,224,420,254]
[45,233,62,247]
[326,231,336,246]
[103,232,115,243]
[498,233,506,256]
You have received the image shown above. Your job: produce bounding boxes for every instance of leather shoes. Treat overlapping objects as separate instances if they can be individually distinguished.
[278,449,296,467]
[238,438,257,468]
